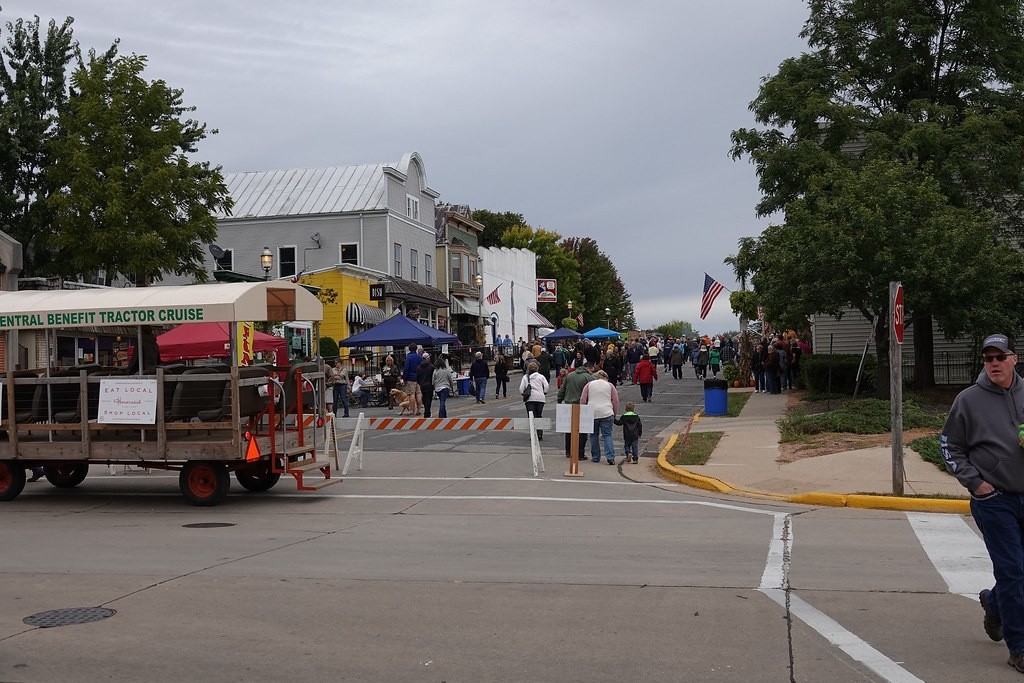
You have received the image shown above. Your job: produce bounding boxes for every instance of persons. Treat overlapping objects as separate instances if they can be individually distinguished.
[496,334,739,403]
[470,351,489,404]
[520,362,550,440]
[332,358,351,417]
[942,334,1024,675]
[128,326,159,373]
[494,355,508,399]
[751,326,811,394]
[352,372,371,407]
[557,362,595,461]
[382,355,399,409]
[310,355,334,413]
[401,343,452,418]
[614,402,642,464]
[303,356,311,362]
[580,371,619,466]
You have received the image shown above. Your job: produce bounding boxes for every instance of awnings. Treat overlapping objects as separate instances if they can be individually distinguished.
[528,309,554,327]
[346,303,386,325]
[386,276,452,321]
[451,295,490,317]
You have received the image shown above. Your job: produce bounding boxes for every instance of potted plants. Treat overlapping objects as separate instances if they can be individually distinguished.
[724,366,757,388]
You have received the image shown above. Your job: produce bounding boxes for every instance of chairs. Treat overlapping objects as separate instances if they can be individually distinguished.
[0,361,318,441]
[345,384,460,409]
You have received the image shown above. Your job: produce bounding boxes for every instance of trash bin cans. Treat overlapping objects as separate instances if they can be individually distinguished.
[704,378,728,415]
[457,377,471,395]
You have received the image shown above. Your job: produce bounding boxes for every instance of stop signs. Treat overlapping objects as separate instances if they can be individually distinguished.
[893,285,904,345]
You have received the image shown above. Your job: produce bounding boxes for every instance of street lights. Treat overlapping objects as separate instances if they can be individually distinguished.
[604,307,611,330]
[567,300,573,319]
[261,246,274,334]
[474,273,482,345]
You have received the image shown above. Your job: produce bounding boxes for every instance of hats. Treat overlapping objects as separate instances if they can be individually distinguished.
[311,355,323,362]
[541,348,546,352]
[981,333,1016,355]
[422,353,431,359]
[475,351,483,358]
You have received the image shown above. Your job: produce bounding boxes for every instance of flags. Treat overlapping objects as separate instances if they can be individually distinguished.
[486,289,500,305]
[577,314,583,325]
[701,274,724,320]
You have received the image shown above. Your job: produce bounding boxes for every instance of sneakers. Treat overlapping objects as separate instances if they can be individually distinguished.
[1007,651,1024,674]
[978,589,1003,642]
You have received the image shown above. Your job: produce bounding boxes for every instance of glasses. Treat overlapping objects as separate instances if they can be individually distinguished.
[982,353,1014,362]
[335,362,339,365]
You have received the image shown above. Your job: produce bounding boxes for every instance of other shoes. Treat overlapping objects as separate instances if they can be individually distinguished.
[476,401,480,404]
[713,376,716,379]
[579,456,587,461]
[503,396,506,399]
[495,394,499,399]
[626,453,633,462]
[342,414,349,417]
[702,378,706,381]
[632,459,638,464]
[699,375,703,380]
[607,459,615,465]
[479,400,486,404]
[389,406,393,410]
[591,459,600,463]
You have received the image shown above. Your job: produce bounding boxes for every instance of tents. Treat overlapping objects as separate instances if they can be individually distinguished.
[543,327,583,353]
[338,312,462,372]
[583,327,620,342]
[155,323,290,378]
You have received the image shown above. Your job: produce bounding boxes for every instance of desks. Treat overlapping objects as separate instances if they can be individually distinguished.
[453,374,471,397]
[360,385,385,399]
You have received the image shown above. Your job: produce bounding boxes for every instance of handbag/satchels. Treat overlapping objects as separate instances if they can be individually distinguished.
[521,373,531,402]
[503,376,510,382]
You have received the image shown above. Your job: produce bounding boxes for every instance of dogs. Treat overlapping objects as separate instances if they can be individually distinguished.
[389,388,410,415]
